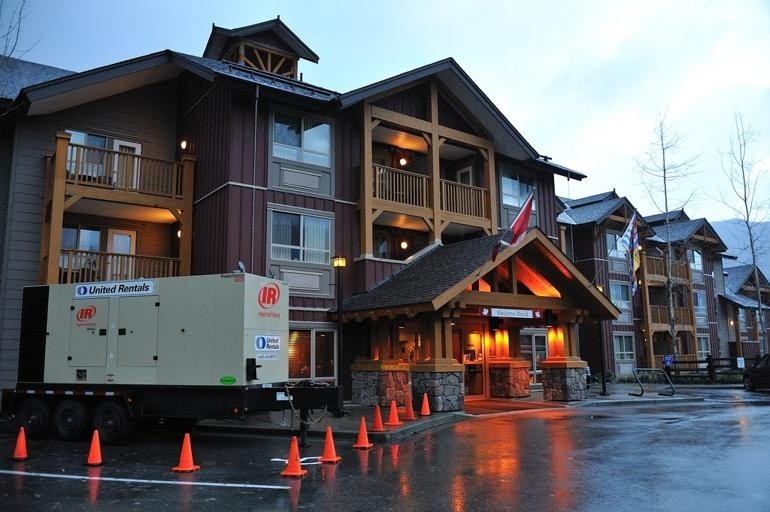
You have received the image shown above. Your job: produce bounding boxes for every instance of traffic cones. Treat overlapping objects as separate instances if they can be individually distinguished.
[357,449,368,473]
[13,428,28,460]
[353,417,373,449]
[318,426,341,464]
[177,472,195,509]
[13,462,25,494]
[280,436,308,478]
[323,464,336,481]
[402,399,416,421]
[172,433,201,473]
[391,443,399,465]
[285,479,301,510]
[420,392,430,416]
[87,430,102,466]
[384,400,402,426]
[87,467,100,502]
[370,404,386,432]
[376,447,384,464]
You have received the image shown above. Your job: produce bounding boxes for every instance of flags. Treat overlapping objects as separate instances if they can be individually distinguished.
[491,193,535,263]
[616,211,643,298]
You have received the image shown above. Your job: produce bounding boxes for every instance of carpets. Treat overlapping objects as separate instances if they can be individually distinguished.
[461,398,567,415]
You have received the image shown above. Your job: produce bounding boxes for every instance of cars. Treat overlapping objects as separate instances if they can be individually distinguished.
[742,353,768,392]
